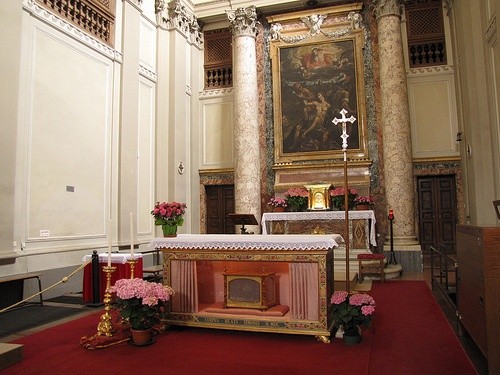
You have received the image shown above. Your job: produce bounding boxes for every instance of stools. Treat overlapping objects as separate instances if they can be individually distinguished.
[357,254,385,284]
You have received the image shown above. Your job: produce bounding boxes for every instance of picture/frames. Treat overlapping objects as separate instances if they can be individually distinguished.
[277,38,363,157]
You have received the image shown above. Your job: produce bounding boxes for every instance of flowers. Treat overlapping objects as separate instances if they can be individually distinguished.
[283,188,310,209]
[329,291,375,335]
[109,278,176,330]
[329,187,358,207]
[353,195,376,206]
[151,201,187,225]
[268,198,288,208]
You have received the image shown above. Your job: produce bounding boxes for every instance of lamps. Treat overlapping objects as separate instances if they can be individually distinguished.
[455,131,471,158]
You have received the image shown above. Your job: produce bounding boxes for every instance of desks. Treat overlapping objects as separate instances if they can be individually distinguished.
[148,234,345,343]
[261,210,377,254]
[82,254,144,303]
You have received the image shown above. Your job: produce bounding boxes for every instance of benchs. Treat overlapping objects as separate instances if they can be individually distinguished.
[143,250,163,282]
[0,271,45,307]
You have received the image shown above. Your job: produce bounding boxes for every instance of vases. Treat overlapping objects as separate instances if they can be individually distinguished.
[343,333,360,346]
[274,207,285,212]
[130,328,151,344]
[341,205,349,210]
[357,204,367,210]
[293,208,302,212]
[162,224,177,236]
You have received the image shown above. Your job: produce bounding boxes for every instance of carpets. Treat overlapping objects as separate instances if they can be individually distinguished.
[0,295,84,339]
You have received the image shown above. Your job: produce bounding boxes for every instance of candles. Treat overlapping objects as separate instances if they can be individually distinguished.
[108,219,111,267]
[130,212,134,259]
